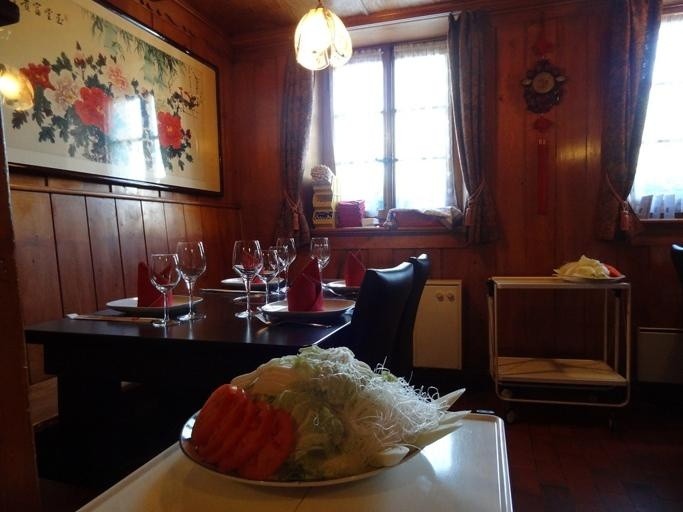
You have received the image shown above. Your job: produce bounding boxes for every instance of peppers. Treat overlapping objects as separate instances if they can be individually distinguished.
[192,379,296,479]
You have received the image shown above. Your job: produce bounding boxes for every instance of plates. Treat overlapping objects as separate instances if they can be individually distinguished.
[325,280,364,294]
[105,294,203,314]
[553,272,626,284]
[221,276,284,289]
[176,406,443,495]
[261,298,356,322]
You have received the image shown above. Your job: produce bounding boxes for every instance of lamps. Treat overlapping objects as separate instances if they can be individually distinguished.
[293,0,352,71]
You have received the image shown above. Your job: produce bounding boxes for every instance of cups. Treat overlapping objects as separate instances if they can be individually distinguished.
[276,237,297,294]
[253,250,280,311]
[268,246,287,295]
[176,240,208,322]
[148,253,180,327]
[232,239,262,318]
[310,237,331,294]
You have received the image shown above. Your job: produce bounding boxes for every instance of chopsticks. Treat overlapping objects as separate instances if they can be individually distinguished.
[74,315,156,323]
[209,289,267,294]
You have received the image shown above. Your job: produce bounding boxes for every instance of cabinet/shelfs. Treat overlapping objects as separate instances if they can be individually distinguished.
[413,280,463,368]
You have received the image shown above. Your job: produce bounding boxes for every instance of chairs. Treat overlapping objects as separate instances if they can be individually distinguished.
[672,242,683,288]
[344,262,413,385]
[409,253,432,317]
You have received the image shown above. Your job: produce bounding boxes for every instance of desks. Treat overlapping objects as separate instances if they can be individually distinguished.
[488,277,631,407]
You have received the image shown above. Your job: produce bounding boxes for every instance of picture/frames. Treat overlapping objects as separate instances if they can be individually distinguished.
[1,0,224,198]
